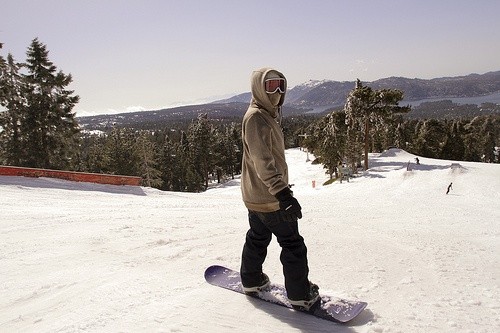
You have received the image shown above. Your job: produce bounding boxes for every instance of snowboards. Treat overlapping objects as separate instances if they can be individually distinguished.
[204,264,368,323]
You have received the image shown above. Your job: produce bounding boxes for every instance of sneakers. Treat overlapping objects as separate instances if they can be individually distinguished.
[288,283,320,306]
[243,273,270,291]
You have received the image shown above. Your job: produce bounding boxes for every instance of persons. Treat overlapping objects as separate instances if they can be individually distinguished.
[446,183,452,194]
[414,158,420,165]
[240,68,321,311]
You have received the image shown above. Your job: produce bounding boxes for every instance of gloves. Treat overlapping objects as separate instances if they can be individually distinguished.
[275,188,303,223]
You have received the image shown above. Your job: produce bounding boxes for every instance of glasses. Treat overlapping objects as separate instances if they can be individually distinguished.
[263,77,287,95]
